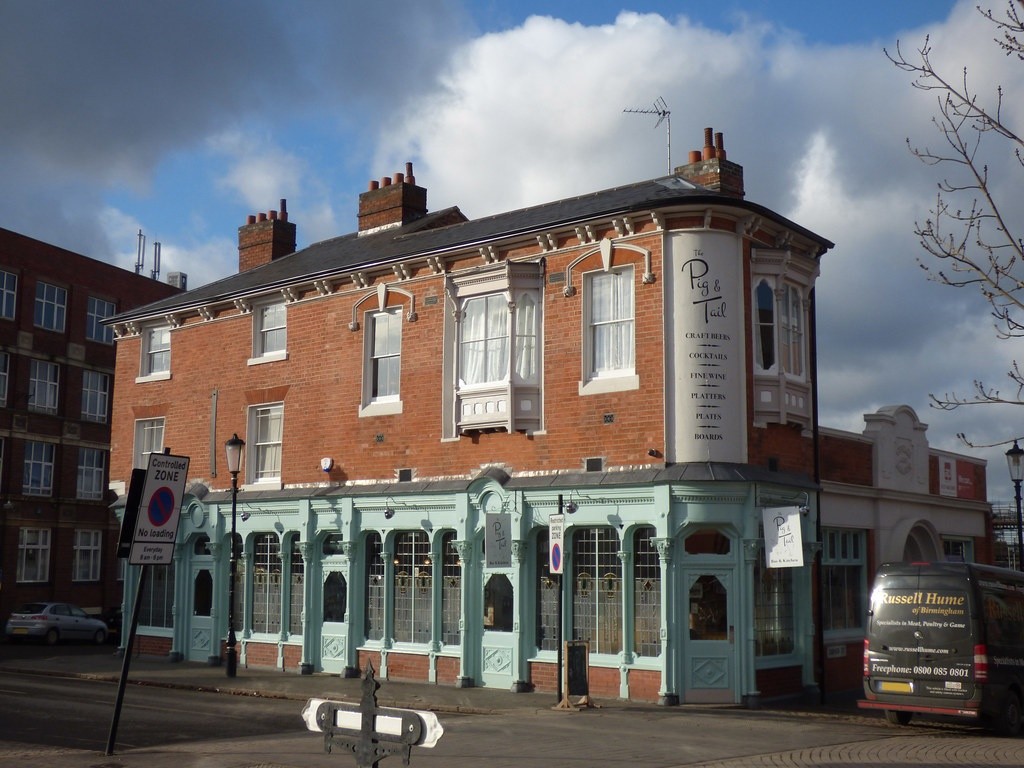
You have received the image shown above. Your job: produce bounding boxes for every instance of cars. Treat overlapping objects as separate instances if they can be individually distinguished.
[105,605,122,641]
[5,602,109,645]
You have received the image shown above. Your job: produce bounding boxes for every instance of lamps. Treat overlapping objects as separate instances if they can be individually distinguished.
[565,488,603,514]
[241,501,269,522]
[384,495,416,519]
[781,489,809,516]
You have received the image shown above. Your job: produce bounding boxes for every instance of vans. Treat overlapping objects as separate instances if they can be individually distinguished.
[855,560,1024,735]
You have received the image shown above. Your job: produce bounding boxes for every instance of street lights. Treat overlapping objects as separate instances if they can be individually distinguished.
[1005,439,1024,572]
[226,433,246,676]
